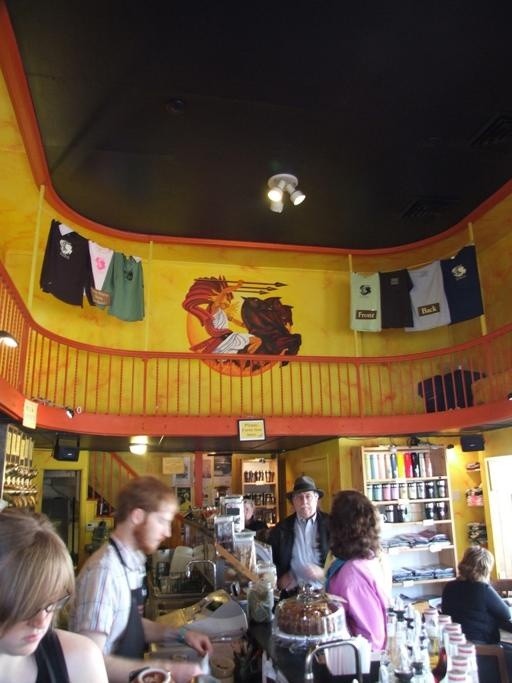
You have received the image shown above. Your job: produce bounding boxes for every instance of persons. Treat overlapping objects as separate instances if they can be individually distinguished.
[322,489,388,682]
[68,475,214,682]
[0,502,109,682]
[243,494,268,530]
[440,544,511,683]
[267,474,334,600]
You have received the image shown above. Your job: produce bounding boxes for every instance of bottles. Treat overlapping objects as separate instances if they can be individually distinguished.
[247,563,277,624]
[364,453,446,523]
[378,591,478,683]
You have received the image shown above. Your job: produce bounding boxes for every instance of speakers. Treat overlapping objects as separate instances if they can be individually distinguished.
[54,446,78,462]
[460,435,484,452]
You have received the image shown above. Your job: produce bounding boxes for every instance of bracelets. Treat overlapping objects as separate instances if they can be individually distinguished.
[176,626,187,643]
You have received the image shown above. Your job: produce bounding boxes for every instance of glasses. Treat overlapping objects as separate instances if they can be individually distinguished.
[18,583,73,623]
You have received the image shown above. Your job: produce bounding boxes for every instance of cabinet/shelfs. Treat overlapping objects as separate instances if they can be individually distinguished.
[348,442,460,611]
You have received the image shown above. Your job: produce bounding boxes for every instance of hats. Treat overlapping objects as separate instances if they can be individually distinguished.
[284,473,325,505]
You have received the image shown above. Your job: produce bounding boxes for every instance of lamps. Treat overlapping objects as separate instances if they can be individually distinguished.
[266,173,305,213]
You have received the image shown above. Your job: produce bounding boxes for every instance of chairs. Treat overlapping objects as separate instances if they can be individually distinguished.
[436,578,511,683]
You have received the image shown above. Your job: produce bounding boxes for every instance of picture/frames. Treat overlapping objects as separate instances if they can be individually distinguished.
[237,418,267,442]
[241,456,280,527]
